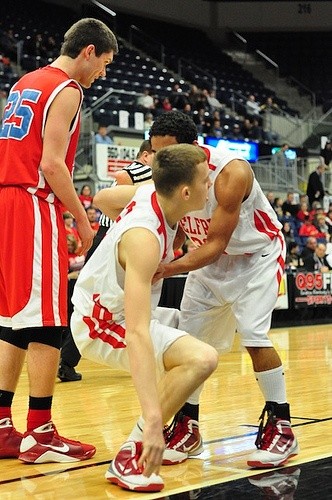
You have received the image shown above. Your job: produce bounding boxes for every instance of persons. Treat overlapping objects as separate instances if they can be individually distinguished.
[0,18,119,464]
[136,88,155,109]
[306,166,325,210]
[266,191,332,272]
[319,140,332,189]
[144,113,155,128]
[71,143,218,494]
[261,97,278,111]
[36,33,58,51]
[151,110,299,466]
[273,144,288,184]
[162,84,269,144]
[63,184,99,271]
[89,125,111,162]
[57,140,156,381]
[0,55,12,73]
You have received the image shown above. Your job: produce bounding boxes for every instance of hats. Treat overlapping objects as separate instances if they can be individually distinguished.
[315,204,323,210]
[0,57,10,65]
[162,103,172,111]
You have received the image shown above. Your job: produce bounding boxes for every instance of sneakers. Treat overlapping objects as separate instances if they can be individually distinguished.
[161,415,205,466]
[0,417,24,458]
[103,441,164,492]
[163,427,172,444]
[247,467,300,498]
[17,420,97,464]
[247,417,300,469]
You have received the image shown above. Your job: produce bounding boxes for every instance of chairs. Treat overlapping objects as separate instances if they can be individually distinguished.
[0,27,300,147]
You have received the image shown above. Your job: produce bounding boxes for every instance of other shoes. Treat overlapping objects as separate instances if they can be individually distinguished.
[57,365,82,381]
